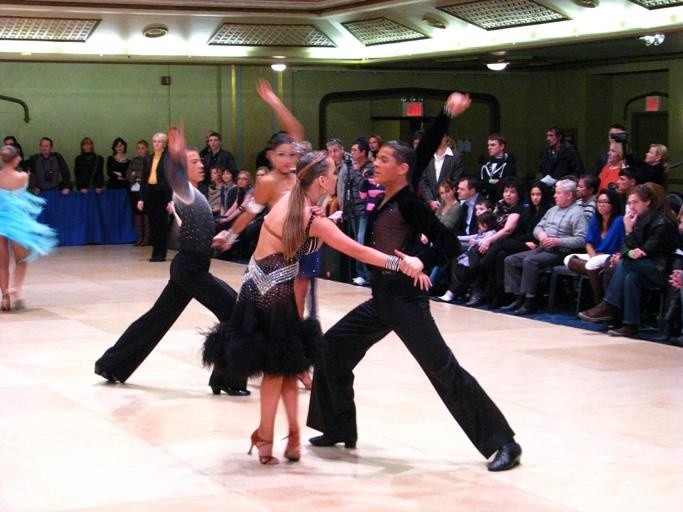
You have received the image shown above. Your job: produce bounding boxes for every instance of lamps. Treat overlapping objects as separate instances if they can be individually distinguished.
[487,63,509,72]
[271,63,287,72]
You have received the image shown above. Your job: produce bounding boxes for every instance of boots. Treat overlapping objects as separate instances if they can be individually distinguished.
[132,214,151,246]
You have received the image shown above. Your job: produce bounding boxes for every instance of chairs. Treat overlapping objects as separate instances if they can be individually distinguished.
[429,264,683,349]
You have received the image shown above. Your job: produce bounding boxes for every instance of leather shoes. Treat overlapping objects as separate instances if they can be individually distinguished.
[149,256,166,262]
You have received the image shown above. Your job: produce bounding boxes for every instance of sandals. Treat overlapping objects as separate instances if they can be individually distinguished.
[297,371,312,390]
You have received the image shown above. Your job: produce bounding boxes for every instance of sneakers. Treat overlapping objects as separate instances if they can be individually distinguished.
[608,325,639,339]
[584,254,611,271]
[438,293,452,302]
[578,301,614,322]
[352,277,366,286]
[464,294,538,314]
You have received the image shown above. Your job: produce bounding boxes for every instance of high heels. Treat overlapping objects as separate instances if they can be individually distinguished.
[309,432,356,449]
[284,430,300,461]
[209,382,251,396]
[95,360,117,383]
[1,299,11,313]
[248,430,279,465]
[488,443,521,471]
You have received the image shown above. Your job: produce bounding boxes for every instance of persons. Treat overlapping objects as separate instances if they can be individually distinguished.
[4,137,149,195]
[212,76,322,392]
[259,125,683,346]
[96,118,250,396]
[308,92,521,472]
[2,145,59,313]
[206,151,432,460]
[138,133,173,263]
[126,140,151,246]
[197,130,270,262]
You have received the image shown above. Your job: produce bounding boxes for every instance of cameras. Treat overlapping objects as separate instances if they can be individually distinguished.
[610,132,630,143]
